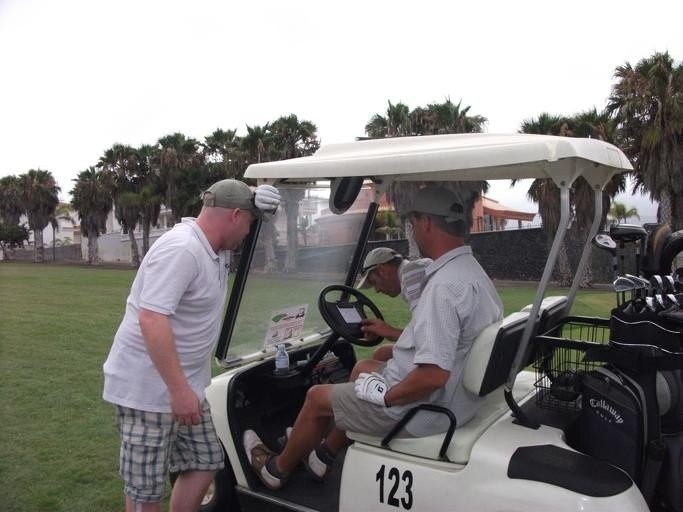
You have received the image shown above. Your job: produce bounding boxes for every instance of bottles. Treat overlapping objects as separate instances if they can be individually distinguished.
[275,345,289,375]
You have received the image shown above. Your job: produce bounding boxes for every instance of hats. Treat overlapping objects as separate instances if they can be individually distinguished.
[200,178,269,224]
[355,245,403,291]
[397,185,465,224]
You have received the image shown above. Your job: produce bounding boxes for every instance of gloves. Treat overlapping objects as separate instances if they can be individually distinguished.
[252,184,282,213]
[351,369,391,409]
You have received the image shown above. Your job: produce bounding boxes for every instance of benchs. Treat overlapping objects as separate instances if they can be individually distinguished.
[341,294,568,462]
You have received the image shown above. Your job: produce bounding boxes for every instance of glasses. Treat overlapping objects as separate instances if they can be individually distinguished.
[359,263,377,277]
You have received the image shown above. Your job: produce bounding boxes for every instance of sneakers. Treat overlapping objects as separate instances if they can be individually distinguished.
[284,425,332,484]
[240,428,289,491]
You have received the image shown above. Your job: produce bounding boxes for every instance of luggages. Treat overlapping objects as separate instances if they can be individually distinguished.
[578,295,683,512]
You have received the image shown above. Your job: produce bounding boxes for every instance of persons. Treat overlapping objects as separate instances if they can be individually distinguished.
[349,248,434,382]
[241,187,503,488]
[103,179,281,512]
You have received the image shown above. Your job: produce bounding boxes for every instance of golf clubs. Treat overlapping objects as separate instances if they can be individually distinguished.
[592,223,683,315]
